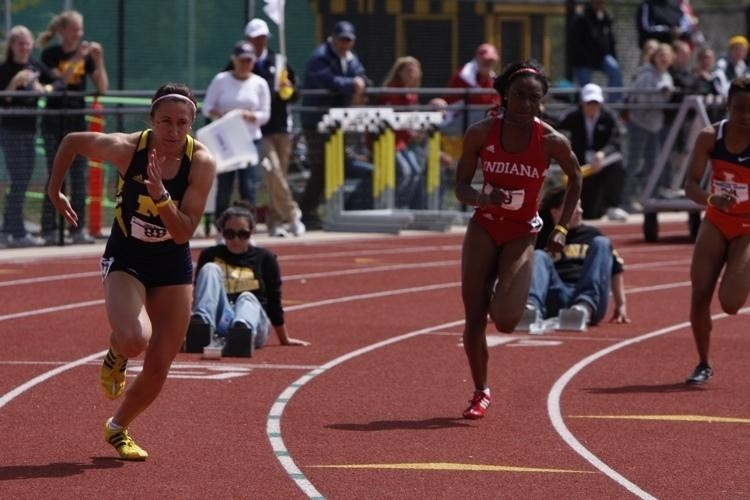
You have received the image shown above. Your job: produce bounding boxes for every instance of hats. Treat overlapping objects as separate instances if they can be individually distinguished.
[476,43,499,62]
[580,83,604,103]
[729,36,748,48]
[245,18,269,39]
[233,41,256,61]
[334,21,358,40]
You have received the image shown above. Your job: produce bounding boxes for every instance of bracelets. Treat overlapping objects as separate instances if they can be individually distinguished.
[476,192,481,207]
[153,193,171,209]
[707,194,714,208]
[554,225,568,236]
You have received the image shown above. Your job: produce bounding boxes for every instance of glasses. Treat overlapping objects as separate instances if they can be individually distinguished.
[222,228,250,241]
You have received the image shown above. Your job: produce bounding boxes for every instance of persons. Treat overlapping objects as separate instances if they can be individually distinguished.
[185,202,312,356]
[225,17,307,237]
[518,187,631,331]
[446,42,500,102]
[294,19,373,226]
[37,11,108,242]
[627,2,749,204]
[558,82,627,219]
[454,59,584,421]
[374,57,449,207]
[0,26,63,246]
[201,42,273,240]
[564,2,623,103]
[48,84,215,460]
[683,78,750,383]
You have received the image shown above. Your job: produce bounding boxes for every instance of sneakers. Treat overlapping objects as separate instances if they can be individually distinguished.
[463,390,492,419]
[100,346,128,401]
[1,230,95,247]
[289,209,305,236]
[103,416,148,460]
[570,301,592,325]
[190,313,208,324]
[231,318,250,329]
[272,227,287,236]
[525,303,544,319]
[686,362,714,385]
[607,207,628,221]
